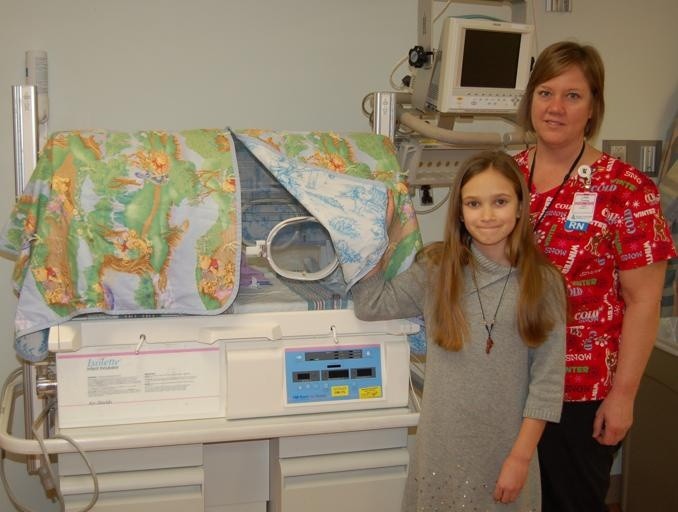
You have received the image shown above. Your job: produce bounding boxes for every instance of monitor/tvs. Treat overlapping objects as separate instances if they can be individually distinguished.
[428,16,536,115]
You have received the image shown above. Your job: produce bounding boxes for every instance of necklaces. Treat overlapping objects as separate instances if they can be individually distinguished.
[467,237,511,356]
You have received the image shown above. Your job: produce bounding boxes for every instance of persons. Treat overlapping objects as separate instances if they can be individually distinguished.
[510,40,678,512]
[348,147,570,512]
